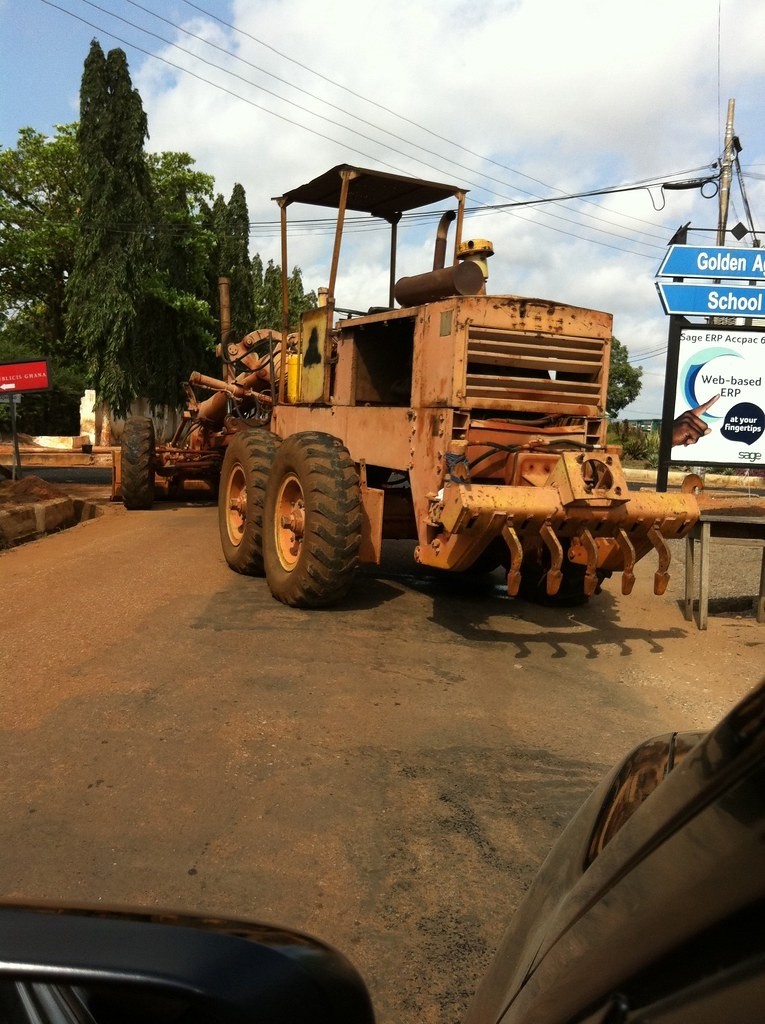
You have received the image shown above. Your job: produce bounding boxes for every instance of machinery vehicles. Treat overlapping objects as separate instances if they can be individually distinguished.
[110,162,765,614]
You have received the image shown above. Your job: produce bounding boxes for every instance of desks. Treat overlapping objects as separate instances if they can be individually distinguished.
[682,513,765,630]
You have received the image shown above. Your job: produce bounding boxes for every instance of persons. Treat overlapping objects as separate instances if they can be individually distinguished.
[672,394,721,448]
